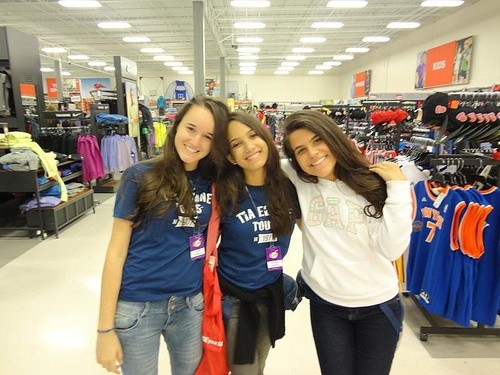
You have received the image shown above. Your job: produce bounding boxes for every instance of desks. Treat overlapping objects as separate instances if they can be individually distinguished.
[1,153,84,239]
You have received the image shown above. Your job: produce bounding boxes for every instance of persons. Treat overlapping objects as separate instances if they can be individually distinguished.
[273,110,414,373]
[215,108,303,371]
[415,51,427,88]
[93,95,217,375]
[458,39,472,82]
[453,45,465,83]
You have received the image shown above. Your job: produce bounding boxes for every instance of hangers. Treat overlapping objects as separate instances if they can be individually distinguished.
[366,88,500,190]
[24,117,128,138]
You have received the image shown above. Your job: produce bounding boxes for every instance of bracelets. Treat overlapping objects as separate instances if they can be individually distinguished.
[96,326,116,334]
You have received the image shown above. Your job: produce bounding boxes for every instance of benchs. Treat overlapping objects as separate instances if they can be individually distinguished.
[25,186,97,239]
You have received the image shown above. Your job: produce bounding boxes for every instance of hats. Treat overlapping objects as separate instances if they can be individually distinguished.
[325,107,368,119]
[422,92,500,145]
[371,102,419,129]
